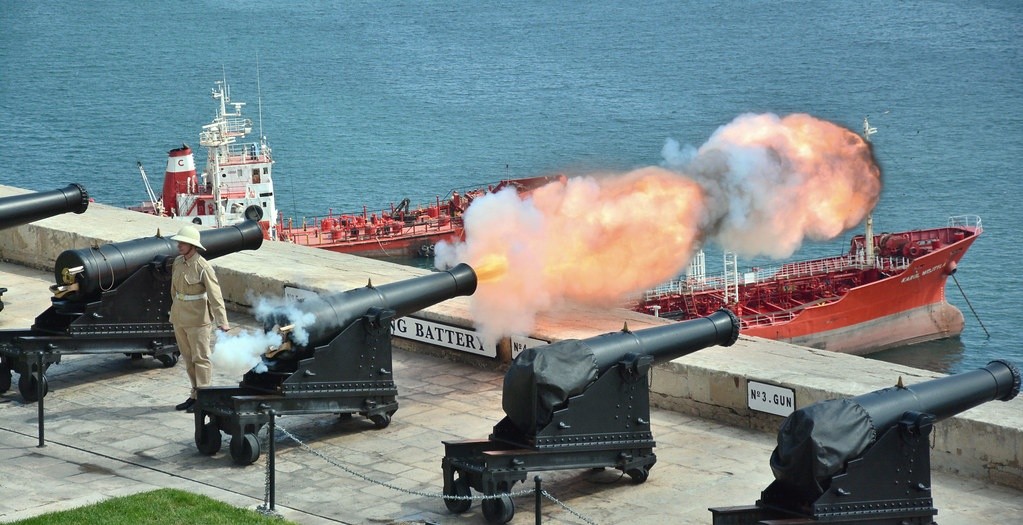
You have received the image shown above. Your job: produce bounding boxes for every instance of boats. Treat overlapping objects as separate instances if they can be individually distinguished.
[122,46,567,270]
[617,114,984,356]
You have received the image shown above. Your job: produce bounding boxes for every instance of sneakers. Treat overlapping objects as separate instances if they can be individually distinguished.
[176,398,195,410]
[187,400,196,413]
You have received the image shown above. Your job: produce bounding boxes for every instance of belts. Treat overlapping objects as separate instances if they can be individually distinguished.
[175,290,207,301]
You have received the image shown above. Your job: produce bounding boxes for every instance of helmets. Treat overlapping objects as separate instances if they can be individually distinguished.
[170,226,206,251]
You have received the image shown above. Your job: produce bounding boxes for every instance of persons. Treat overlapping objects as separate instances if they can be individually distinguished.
[169,227,230,413]
